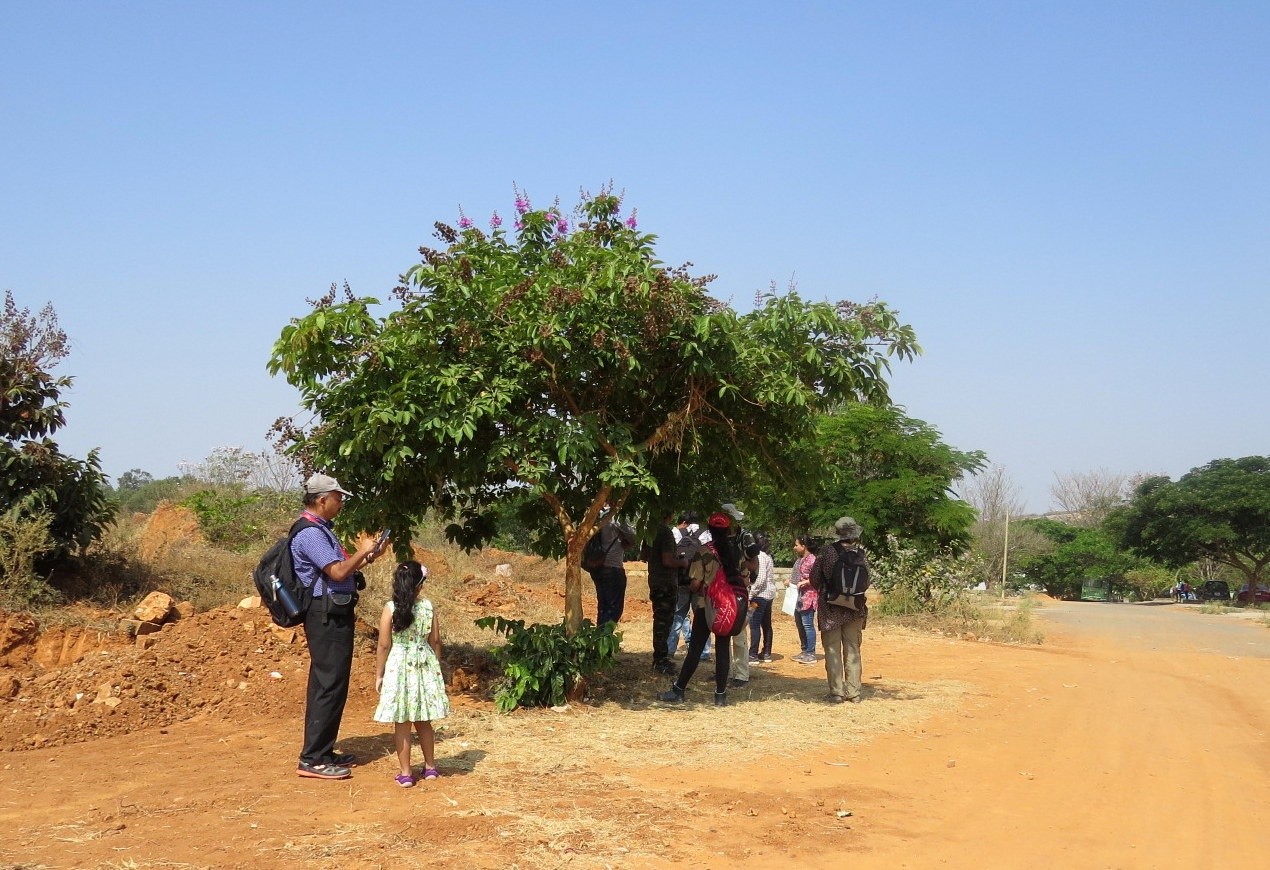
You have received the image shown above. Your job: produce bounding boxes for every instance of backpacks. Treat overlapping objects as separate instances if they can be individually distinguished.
[676,529,708,586]
[820,542,868,611]
[253,520,334,628]
[701,541,748,636]
[581,529,621,571]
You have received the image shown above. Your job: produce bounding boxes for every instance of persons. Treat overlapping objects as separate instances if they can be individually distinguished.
[292,476,390,779]
[375,561,449,788]
[1169,578,1197,602]
[590,498,869,706]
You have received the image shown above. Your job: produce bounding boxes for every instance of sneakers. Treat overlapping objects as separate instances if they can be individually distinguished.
[843,696,860,703]
[791,652,806,661]
[331,755,356,766]
[799,654,818,663]
[732,679,748,689]
[295,761,351,778]
[823,694,843,703]
[656,661,678,676]
[758,653,773,663]
[749,656,759,665]
[725,678,733,688]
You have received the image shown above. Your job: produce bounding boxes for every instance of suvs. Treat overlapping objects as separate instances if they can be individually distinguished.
[1203,580,1230,600]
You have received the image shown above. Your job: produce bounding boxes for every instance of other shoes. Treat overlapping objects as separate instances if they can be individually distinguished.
[421,767,439,780]
[395,774,415,787]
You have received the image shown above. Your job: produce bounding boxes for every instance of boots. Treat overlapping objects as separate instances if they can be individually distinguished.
[715,690,728,706]
[658,681,684,704]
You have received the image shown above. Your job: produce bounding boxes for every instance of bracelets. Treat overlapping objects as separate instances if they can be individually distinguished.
[365,555,374,563]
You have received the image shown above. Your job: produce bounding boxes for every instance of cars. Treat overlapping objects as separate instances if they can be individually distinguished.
[1233,583,1270,602]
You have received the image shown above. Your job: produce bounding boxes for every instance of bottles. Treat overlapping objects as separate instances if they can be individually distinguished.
[678,554,685,574]
[743,602,758,629]
[269,574,300,619]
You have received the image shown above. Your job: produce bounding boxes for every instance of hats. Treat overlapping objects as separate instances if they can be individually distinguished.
[722,504,744,522]
[710,513,730,527]
[835,516,864,540]
[304,474,354,497]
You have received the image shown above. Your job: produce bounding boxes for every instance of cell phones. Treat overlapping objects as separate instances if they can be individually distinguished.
[377,528,391,545]
